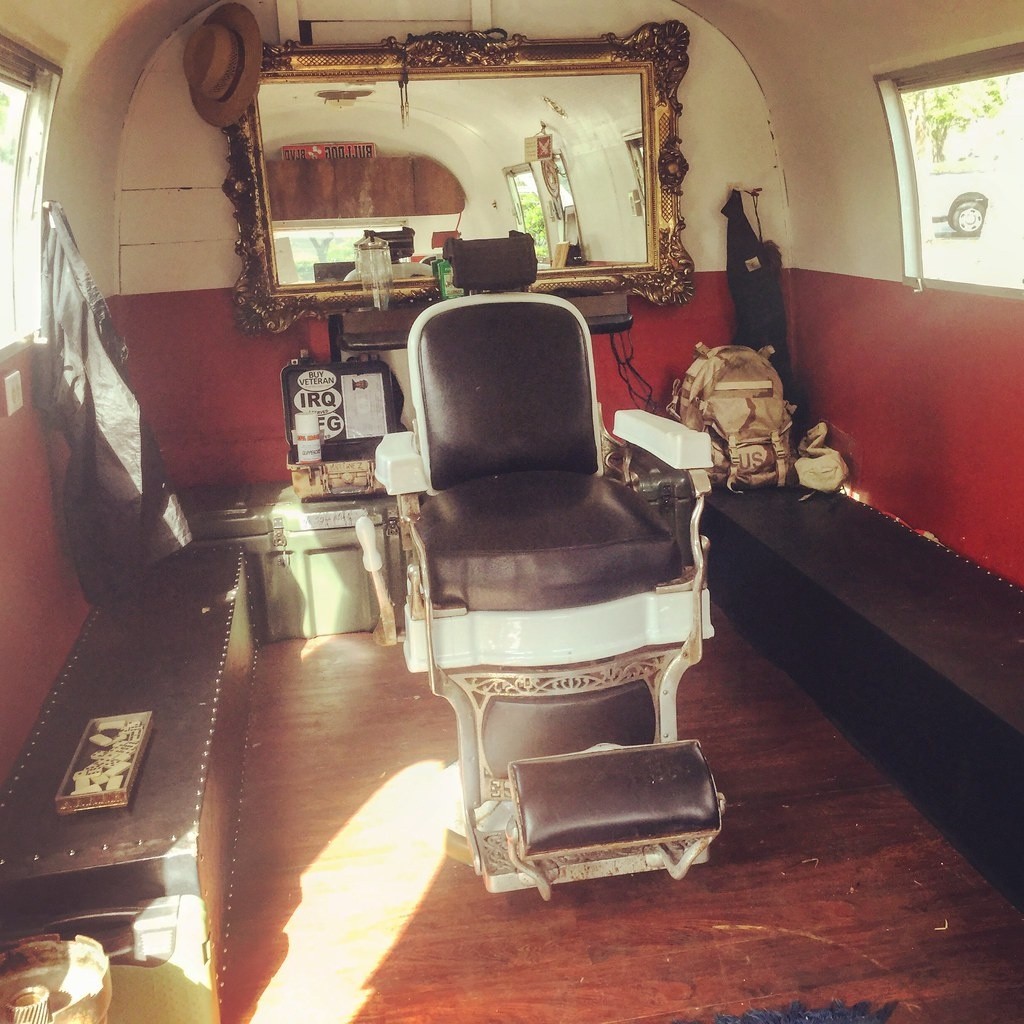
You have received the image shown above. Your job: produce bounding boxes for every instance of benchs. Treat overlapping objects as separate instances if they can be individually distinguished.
[164,443,697,643]
[0,542,263,1024]
[697,481,1024,914]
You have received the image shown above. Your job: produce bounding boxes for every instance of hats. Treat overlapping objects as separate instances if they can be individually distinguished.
[182,2,261,128]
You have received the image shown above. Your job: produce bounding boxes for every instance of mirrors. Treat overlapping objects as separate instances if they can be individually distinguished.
[221,19,696,336]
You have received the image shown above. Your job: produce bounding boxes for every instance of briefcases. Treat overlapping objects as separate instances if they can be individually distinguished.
[281,360,398,501]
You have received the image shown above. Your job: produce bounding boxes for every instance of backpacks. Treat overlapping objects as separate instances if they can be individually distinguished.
[665,342,797,493]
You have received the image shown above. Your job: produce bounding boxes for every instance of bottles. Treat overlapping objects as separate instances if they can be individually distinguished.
[295,413,321,464]
[438,259,464,300]
[431,253,444,277]
[359,230,395,310]
[354,230,370,281]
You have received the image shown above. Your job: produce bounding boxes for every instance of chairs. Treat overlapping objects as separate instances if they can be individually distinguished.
[354,228,729,902]
